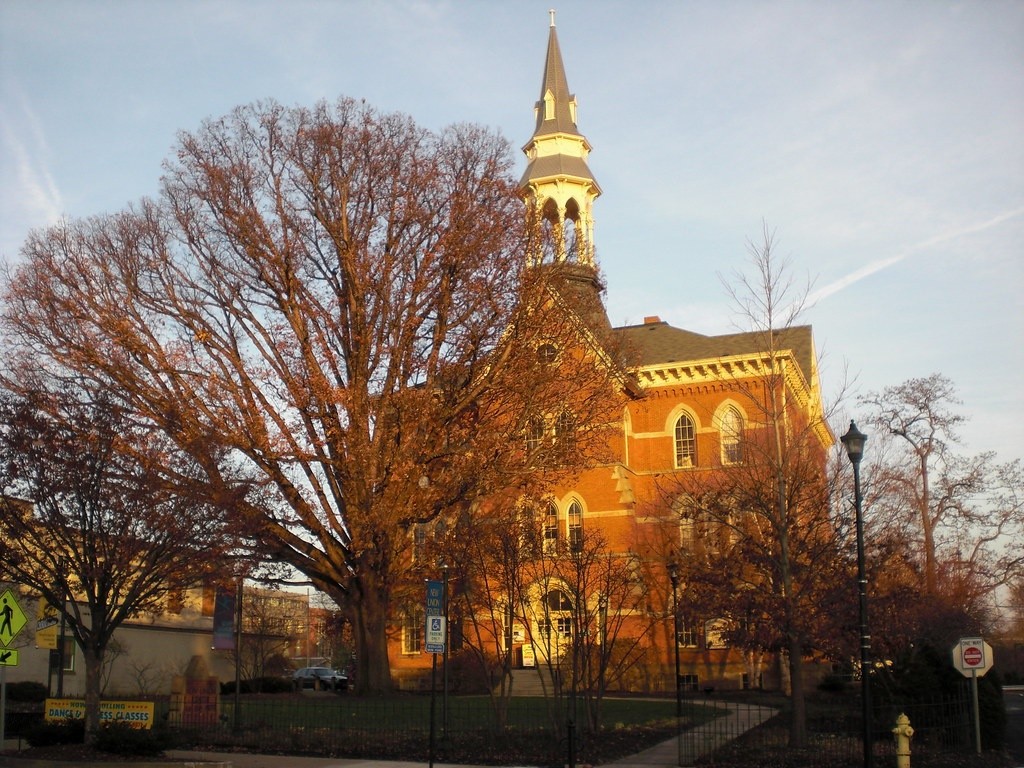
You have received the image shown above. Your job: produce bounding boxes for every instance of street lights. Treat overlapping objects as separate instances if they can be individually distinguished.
[838,418,874,768]
[664,550,685,717]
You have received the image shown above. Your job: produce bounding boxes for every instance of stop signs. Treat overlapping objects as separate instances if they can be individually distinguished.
[964,647,983,666]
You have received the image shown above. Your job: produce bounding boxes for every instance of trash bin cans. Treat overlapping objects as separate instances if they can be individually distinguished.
[522,644,535,667]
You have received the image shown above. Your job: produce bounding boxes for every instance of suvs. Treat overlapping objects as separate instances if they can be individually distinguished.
[290,667,348,693]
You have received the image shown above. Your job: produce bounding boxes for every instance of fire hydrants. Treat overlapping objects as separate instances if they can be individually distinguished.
[890,713,914,768]
